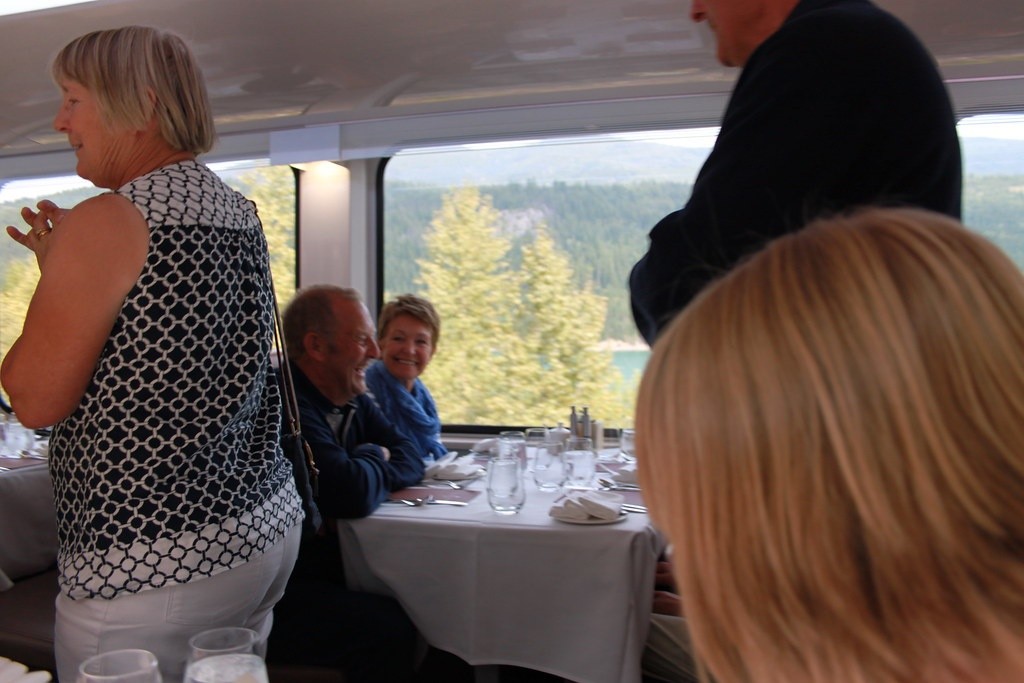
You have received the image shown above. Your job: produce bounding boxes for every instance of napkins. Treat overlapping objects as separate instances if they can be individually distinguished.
[472,437,502,454]
[422,452,484,478]
[548,488,625,521]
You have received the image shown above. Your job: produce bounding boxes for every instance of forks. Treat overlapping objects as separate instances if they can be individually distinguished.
[384,495,433,506]
[17,452,49,460]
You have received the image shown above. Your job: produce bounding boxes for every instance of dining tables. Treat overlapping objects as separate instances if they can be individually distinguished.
[0,456,58,587]
[335,443,664,683]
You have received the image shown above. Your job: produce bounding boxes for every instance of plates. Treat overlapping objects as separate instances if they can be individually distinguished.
[433,475,479,481]
[547,507,630,524]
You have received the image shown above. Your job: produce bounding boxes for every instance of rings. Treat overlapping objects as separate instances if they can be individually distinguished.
[37,229,49,239]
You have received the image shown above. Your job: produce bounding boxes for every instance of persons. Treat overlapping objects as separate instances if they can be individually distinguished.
[358,294,448,461]
[275,283,472,683]
[627,0,962,355]
[641,561,701,683]
[0,26,306,683]
[633,206,1024,683]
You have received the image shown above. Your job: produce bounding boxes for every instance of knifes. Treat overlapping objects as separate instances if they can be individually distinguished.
[384,498,469,506]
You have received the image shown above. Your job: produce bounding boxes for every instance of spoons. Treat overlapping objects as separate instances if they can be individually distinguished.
[596,476,643,494]
[21,450,48,457]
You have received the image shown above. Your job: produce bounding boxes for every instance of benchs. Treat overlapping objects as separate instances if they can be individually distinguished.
[0,565,60,678]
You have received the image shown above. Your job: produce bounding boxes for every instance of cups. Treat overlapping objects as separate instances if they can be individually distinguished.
[182,627,269,682]
[616,419,637,457]
[487,428,595,516]
[0,411,35,454]
[76,649,163,683]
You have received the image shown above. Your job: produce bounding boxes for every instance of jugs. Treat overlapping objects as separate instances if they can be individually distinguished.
[541,423,571,456]
